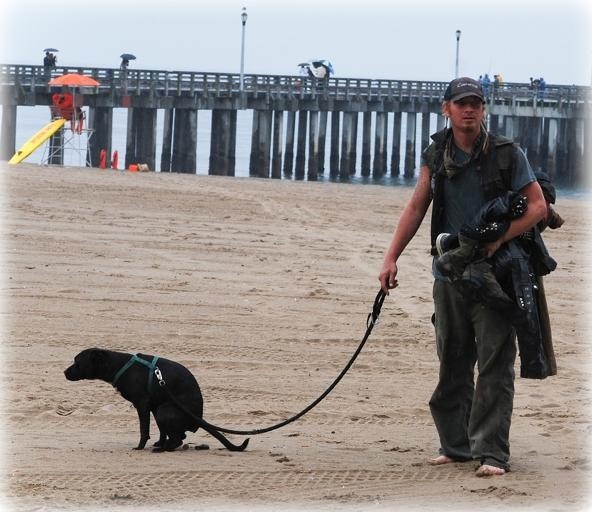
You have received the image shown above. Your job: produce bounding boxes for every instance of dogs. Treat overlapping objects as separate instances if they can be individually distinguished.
[63,347,251,453]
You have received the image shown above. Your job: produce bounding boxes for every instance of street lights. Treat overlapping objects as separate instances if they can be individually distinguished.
[455,30,461,79]
[240,6,248,90]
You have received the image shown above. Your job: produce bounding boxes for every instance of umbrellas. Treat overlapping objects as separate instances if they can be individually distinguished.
[312,59,334,74]
[46,73,102,108]
[43,48,59,52]
[120,53,137,60]
[297,63,311,66]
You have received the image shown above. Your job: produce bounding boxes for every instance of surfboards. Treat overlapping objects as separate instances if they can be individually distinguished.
[8,119,67,164]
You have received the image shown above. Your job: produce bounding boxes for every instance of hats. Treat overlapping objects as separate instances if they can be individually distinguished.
[445,76,484,104]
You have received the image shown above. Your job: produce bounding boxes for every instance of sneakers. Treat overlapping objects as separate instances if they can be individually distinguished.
[435,232,513,312]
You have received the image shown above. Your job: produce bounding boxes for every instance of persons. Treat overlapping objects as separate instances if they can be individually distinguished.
[316,65,330,86]
[379,77,550,477]
[307,67,315,76]
[529,77,547,98]
[44,51,56,82]
[119,61,129,79]
[478,74,504,97]
[300,66,308,93]
